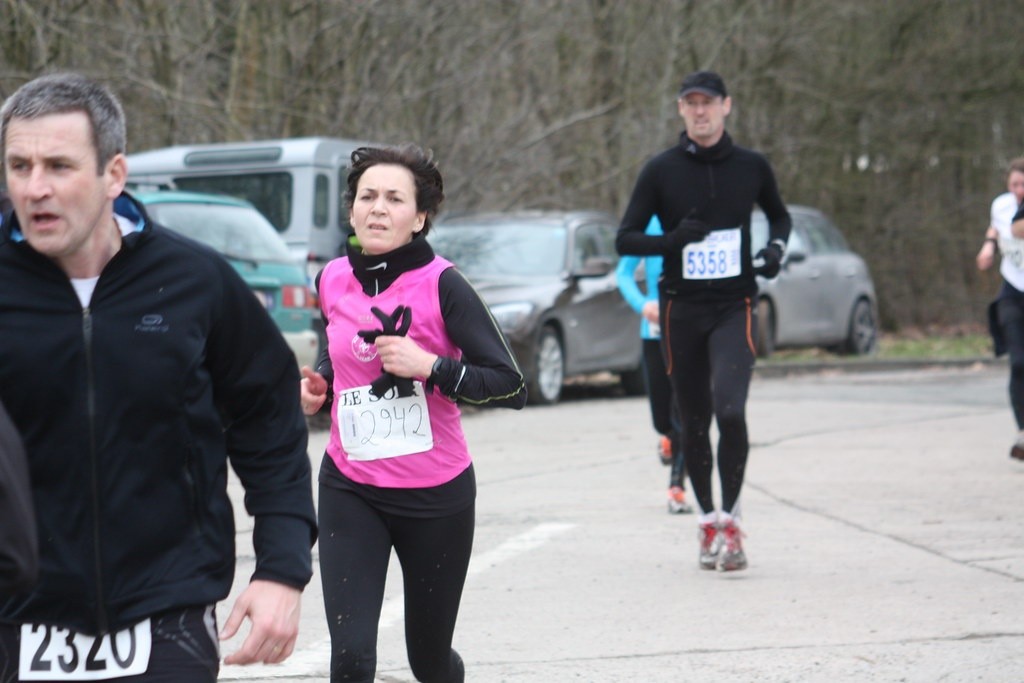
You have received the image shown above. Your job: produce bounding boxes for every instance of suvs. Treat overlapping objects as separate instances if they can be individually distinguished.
[135,187,323,375]
[124,136,384,293]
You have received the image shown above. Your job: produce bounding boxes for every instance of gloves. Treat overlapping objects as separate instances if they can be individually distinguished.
[358,305,418,398]
[754,242,784,279]
[666,207,709,253]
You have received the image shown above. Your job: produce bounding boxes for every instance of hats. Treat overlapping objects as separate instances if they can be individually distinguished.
[680,71,726,99]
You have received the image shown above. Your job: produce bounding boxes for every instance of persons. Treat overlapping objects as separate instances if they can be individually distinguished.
[1,74,319,683]
[301,144,528,683]
[976,158,1024,461]
[611,71,792,579]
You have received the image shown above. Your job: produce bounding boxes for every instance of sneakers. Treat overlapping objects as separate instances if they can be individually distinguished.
[658,435,672,465]
[697,519,716,570]
[667,487,691,513]
[717,520,747,571]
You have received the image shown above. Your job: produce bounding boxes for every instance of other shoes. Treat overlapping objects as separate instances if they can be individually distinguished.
[1010,443,1024,460]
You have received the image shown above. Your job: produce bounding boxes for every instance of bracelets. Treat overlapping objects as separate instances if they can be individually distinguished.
[769,237,786,252]
[983,238,998,243]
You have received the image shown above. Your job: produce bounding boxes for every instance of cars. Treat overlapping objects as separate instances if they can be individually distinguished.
[751,202,881,359]
[427,210,649,404]
